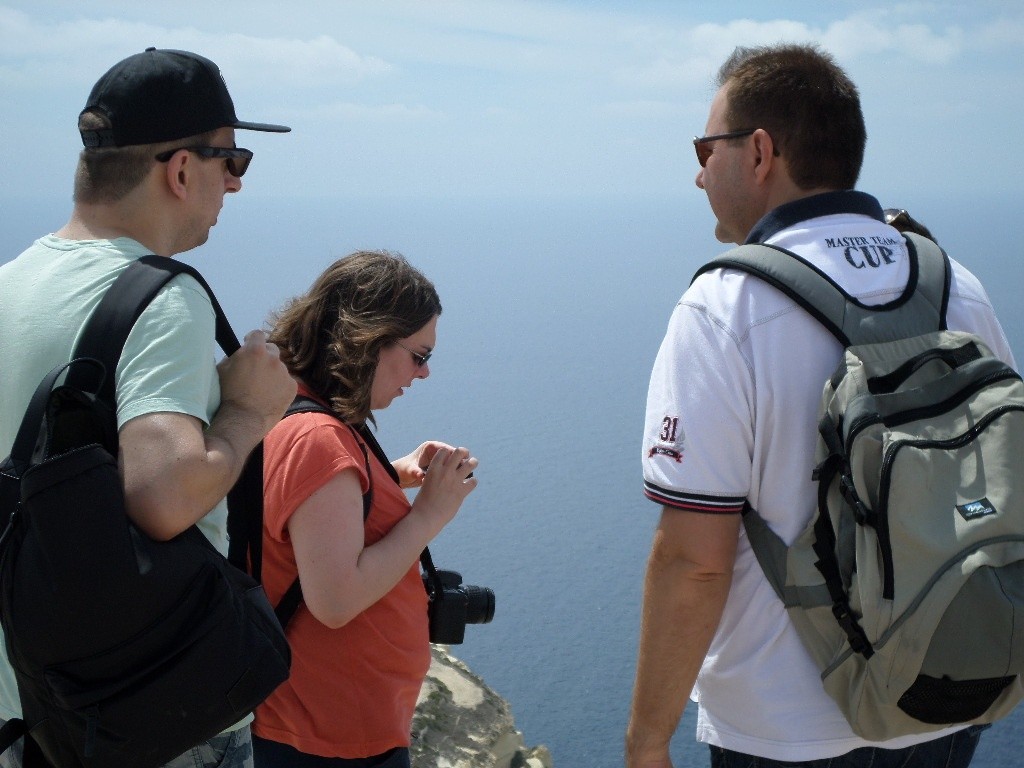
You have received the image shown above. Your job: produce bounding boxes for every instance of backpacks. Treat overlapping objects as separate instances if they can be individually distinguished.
[0,255,292,768]
[687,229,1023,739]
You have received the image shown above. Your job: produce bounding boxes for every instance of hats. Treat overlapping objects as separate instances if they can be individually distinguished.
[78,47,291,148]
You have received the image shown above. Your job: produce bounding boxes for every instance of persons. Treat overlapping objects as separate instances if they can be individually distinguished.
[626,48,1024,768]
[0,48,299,768]
[233,251,479,768]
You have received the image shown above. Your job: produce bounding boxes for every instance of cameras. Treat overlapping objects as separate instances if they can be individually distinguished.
[420,569,495,645]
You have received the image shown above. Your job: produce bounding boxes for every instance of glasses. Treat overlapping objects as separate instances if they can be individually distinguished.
[692,129,781,167]
[156,145,253,177]
[391,339,432,366]
